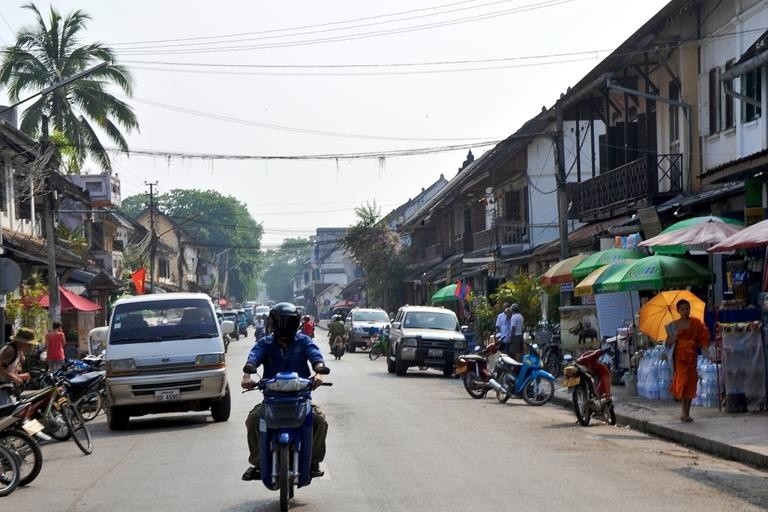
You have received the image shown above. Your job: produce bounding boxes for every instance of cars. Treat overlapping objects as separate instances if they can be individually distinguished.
[93,288,253,427]
[245,299,307,319]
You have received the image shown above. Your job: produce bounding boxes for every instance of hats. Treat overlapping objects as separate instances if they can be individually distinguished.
[9,328,39,346]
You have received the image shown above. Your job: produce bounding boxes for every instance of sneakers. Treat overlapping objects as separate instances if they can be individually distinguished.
[310,468,325,477]
[241,465,261,480]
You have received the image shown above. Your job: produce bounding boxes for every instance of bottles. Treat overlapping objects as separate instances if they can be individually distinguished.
[633,347,722,409]
[716,299,750,336]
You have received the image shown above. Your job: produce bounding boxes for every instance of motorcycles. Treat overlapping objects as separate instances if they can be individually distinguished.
[298,321,318,340]
[365,326,389,360]
[456,333,618,426]
[240,362,333,511]
[1,347,105,493]
[327,324,346,359]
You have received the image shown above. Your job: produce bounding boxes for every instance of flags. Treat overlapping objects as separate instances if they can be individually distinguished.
[454,279,471,301]
[129,268,147,295]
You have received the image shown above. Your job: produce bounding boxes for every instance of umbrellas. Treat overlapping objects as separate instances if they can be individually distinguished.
[333,300,356,306]
[430,284,470,313]
[572,257,643,351]
[541,252,594,286]
[23,280,102,316]
[707,220,768,293]
[638,288,708,344]
[571,245,648,280]
[599,252,716,296]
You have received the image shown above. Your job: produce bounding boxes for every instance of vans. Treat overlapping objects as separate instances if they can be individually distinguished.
[330,305,352,320]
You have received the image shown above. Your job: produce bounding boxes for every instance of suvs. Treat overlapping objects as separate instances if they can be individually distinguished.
[346,307,393,350]
[387,299,470,378]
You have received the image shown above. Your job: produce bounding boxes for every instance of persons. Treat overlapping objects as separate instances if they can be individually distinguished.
[665,299,710,423]
[501,308,512,355]
[0,327,41,411]
[239,302,328,479]
[496,302,511,353]
[508,303,525,362]
[44,320,67,375]
[252,307,347,356]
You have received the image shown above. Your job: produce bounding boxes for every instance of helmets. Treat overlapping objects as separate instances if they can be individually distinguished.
[270,302,301,340]
